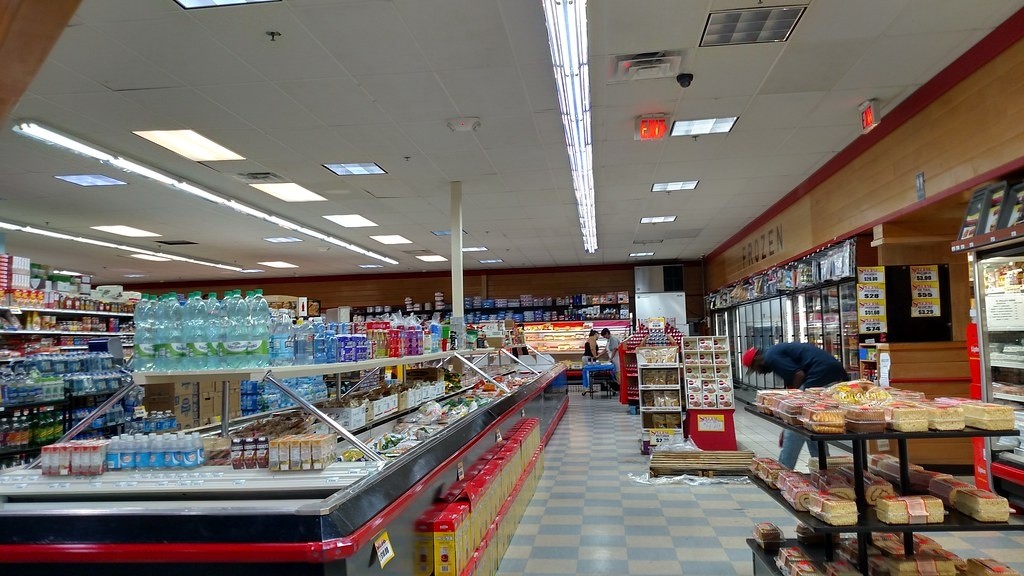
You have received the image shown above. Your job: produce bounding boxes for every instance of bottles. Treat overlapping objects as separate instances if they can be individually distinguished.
[134,289,337,374]
[0,350,204,473]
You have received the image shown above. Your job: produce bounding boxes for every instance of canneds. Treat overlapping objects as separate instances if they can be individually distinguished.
[40,315,91,331]
[78,297,130,313]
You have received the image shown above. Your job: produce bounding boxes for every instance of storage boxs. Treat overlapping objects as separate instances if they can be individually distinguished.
[413,417,529,576]
[269,368,445,471]
[434,417,546,576]
[148,378,240,432]
[591,292,629,318]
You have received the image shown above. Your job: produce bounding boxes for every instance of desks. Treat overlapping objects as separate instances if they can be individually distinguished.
[582,365,619,399]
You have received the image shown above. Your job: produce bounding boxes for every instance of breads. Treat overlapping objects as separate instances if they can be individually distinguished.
[754,382,1015,576]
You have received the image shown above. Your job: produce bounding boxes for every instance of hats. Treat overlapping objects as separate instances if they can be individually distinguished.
[742,346,760,377]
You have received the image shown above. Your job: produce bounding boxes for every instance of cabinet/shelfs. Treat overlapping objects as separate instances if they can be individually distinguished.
[741,405,1023,576]
[680,337,734,410]
[0,307,138,462]
[635,348,685,434]
[619,322,688,404]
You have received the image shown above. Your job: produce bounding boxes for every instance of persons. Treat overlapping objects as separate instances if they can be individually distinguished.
[743,340,852,469]
[595,328,622,384]
[582,329,600,393]
